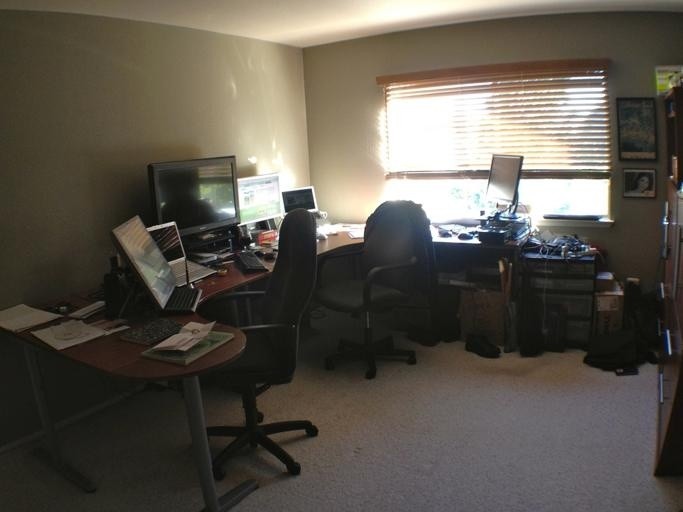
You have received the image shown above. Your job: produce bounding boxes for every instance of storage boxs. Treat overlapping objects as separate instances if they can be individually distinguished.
[595,282,624,332]
[460,261,512,340]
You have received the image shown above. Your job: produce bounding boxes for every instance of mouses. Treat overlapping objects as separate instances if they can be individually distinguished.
[317,232,327,239]
[458,231,475,239]
[255,250,267,258]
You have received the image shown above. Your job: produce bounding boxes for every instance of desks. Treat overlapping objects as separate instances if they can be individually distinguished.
[0,212,533,512]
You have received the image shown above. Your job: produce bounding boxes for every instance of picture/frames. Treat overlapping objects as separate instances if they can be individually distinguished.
[616,95,657,161]
[623,167,656,198]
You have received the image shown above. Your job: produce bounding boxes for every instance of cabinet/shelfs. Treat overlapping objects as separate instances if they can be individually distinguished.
[663,90,682,188]
[652,181,683,477]
[520,243,602,357]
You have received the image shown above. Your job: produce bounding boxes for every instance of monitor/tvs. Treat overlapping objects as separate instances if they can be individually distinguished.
[282,185,319,217]
[237,171,289,231]
[109,214,178,312]
[145,154,241,240]
[483,153,526,218]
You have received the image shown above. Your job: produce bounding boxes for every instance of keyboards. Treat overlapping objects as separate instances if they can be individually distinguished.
[156,288,203,315]
[238,250,268,274]
[440,223,465,234]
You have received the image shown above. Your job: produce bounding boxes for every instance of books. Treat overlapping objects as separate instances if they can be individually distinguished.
[139,330,235,367]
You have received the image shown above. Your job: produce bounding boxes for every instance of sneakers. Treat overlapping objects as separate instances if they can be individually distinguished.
[465,330,502,359]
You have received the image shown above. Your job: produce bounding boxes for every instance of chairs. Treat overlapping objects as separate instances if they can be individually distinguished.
[206,210,320,480]
[317,199,432,380]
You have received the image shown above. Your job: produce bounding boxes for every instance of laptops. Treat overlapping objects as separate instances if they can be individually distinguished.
[144,220,215,288]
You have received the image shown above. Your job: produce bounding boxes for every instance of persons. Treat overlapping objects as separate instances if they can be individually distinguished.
[627,173,652,194]
